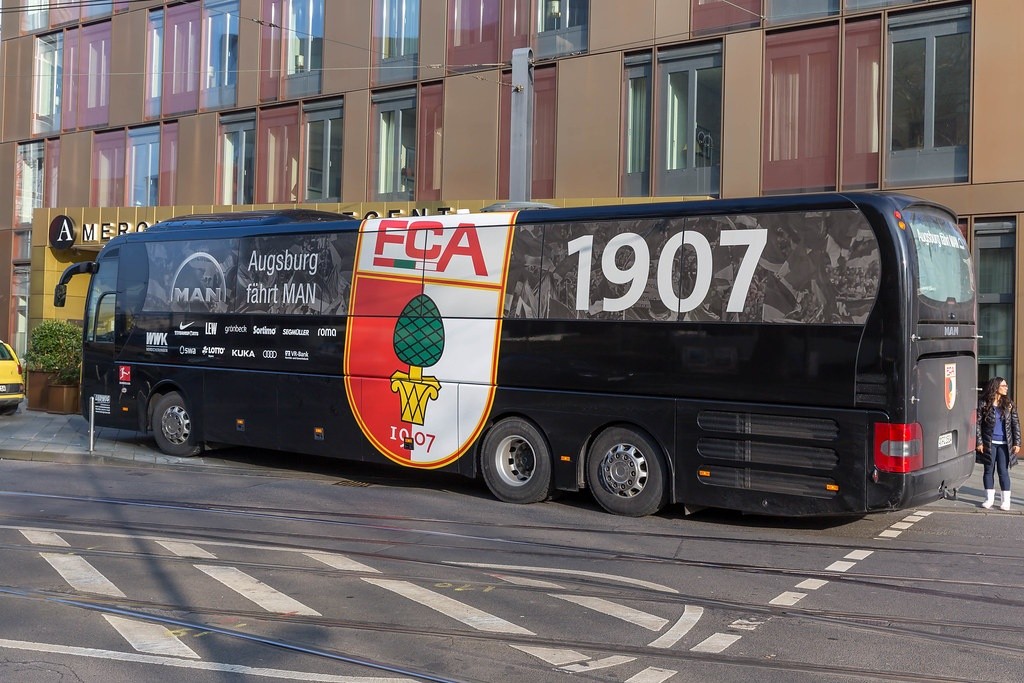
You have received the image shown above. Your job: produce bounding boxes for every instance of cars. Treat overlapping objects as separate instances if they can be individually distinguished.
[0,339,26,416]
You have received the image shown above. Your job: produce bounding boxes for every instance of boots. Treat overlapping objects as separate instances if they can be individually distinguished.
[999,490,1011,510]
[982,488,995,507]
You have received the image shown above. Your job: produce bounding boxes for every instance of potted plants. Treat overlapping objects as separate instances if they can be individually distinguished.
[22,319,83,415]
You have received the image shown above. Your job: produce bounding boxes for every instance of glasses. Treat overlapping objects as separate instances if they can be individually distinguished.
[1001,385,1008,387]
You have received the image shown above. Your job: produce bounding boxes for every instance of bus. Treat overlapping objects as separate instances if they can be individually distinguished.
[54,189,985,520]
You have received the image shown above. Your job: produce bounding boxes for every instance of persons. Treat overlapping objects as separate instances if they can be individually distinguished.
[976,377,1022,512]
